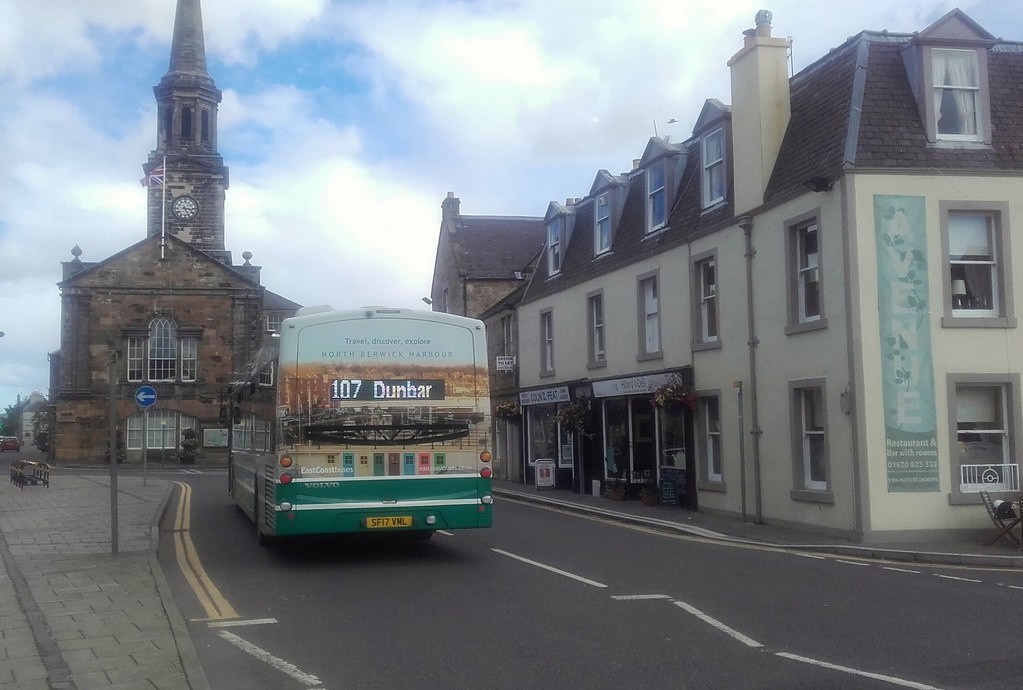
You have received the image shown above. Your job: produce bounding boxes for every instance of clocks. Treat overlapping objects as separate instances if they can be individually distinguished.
[172,196,199,220]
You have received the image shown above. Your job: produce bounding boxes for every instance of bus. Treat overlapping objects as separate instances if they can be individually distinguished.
[228,306,495,545]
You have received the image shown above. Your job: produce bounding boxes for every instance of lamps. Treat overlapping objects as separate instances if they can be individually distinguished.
[802,176,833,192]
[951,280,967,309]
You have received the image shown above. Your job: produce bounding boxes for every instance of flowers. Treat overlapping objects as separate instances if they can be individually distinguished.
[649,381,690,409]
[549,399,592,435]
[493,400,521,421]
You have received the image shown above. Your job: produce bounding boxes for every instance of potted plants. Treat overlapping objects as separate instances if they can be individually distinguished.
[178,427,203,465]
[106,427,128,464]
[640,482,660,505]
[609,476,627,500]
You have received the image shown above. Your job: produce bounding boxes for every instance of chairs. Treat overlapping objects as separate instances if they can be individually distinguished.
[980,491,1022,547]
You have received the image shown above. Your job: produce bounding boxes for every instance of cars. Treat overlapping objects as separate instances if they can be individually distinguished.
[0,436,21,452]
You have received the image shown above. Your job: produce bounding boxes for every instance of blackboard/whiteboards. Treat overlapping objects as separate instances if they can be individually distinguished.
[659,465,687,506]
